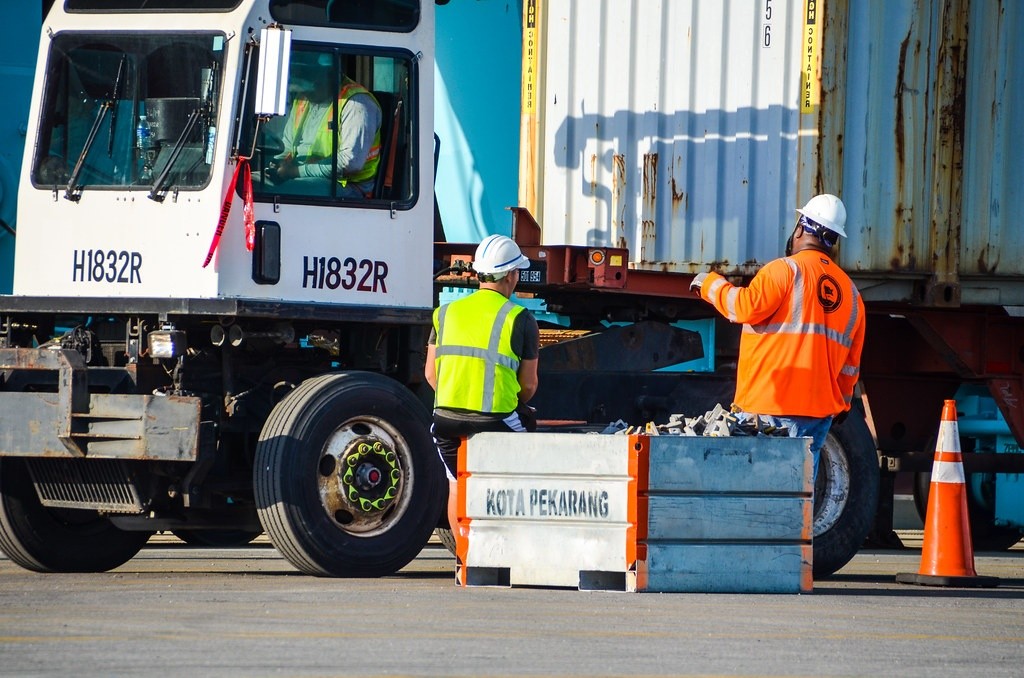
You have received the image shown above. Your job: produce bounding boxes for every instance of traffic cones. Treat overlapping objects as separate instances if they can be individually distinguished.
[895,400,1000,587]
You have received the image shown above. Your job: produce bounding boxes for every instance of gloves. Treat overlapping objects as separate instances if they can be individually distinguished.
[689,272,710,292]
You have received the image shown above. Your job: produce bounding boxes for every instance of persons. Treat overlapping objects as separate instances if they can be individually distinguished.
[424,234,540,538]
[252,55,382,198]
[689,194,865,487]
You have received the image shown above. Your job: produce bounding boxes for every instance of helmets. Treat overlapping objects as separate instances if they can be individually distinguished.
[472,234,530,274]
[796,194,849,239]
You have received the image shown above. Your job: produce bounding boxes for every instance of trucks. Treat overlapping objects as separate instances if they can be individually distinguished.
[1,1,1023,579]
